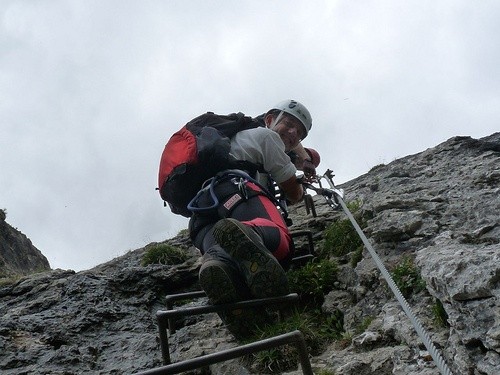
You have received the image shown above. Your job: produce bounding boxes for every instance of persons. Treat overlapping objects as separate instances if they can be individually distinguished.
[187,99,320,338]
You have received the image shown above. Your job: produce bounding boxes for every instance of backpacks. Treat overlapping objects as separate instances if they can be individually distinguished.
[157,110,264,218]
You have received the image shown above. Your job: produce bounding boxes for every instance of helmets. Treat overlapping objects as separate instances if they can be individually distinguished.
[266,99,313,136]
[305,147,321,168]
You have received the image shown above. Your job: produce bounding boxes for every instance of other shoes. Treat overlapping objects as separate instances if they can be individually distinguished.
[198,258,258,339]
[214,218,292,298]
